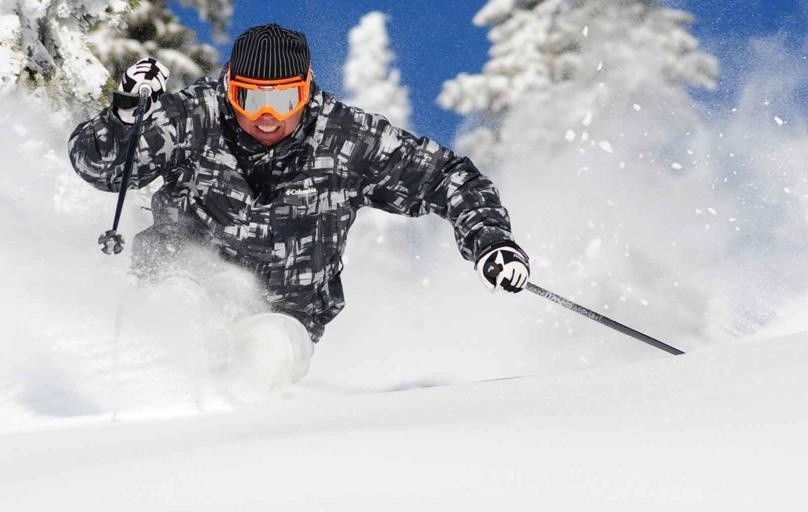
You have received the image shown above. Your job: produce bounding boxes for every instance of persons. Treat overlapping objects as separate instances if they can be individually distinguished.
[67,22,531,406]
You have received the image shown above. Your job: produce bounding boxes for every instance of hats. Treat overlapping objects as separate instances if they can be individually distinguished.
[231,23,311,80]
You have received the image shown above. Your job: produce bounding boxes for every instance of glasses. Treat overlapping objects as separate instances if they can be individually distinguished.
[224,59,313,122]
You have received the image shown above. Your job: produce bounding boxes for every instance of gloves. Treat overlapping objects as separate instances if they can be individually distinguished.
[110,54,170,125]
[476,240,530,294]
